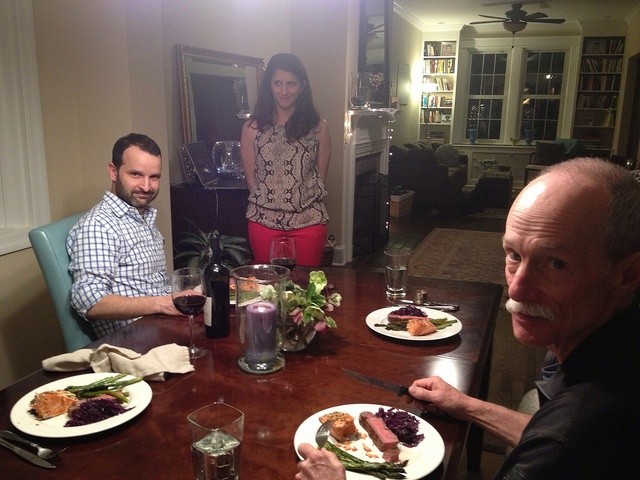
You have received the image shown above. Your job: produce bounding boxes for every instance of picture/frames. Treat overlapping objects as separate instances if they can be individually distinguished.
[274,328,315,351]
[586,38,607,54]
[177,43,266,156]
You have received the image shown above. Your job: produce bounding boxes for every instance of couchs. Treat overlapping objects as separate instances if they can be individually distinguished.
[389,139,468,205]
[524,139,586,185]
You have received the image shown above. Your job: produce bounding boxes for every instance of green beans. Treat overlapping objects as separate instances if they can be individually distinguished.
[323,441,409,480]
[66,372,144,404]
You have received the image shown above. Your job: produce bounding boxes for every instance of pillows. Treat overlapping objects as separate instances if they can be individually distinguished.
[564,142,592,160]
[535,141,563,166]
[435,144,459,167]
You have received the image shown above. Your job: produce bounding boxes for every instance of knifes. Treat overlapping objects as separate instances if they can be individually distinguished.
[400,299,461,312]
[0,436,57,470]
[340,367,431,405]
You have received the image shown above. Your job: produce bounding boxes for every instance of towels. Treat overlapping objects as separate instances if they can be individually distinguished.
[89,344,197,383]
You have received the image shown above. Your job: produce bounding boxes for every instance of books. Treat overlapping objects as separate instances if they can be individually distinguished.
[607,38,625,54]
[425,43,437,56]
[423,59,454,73]
[424,131,445,145]
[579,75,621,91]
[577,133,605,149]
[581,57,622,72]
[422,95,452,108]
[423,77,452,90]
[577,94,617,109]
[421,110,452,123]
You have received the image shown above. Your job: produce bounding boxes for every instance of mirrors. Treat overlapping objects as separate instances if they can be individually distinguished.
[358,1,391,107]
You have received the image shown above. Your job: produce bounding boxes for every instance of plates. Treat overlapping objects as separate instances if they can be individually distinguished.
[193,276,268,308]
[293,403,445,480]
[365,306,463,341]
[9,372,153,439]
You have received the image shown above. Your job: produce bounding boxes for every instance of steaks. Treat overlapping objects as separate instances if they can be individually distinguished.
[359,412,399,463]
[387,314,428,322]
[68,394,119,416]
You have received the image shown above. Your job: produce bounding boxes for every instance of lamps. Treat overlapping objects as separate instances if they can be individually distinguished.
[423,76,437,139]
[504,22,526,48]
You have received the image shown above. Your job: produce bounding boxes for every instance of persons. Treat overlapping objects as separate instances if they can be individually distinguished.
[65,131,204,340]
[295,155,640,480]
[240,53,334,267]
[588,42,604,53]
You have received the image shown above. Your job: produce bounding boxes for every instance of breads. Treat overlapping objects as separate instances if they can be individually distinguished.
[319,411,359,441]
[31,389,79,420]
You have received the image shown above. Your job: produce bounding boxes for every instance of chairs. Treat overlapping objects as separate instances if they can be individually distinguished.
[27,212,95,352]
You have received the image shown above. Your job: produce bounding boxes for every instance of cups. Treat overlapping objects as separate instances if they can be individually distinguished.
[230,78,250,114]
[383,248,411,296]
[230,264,291,374]
[350,71,378,110]
[187,401,245,480]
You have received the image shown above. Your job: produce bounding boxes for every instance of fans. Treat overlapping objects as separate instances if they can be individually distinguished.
[468,4,565,25]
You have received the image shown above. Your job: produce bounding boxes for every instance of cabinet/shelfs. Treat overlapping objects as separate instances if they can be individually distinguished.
[417,38,459,145]
[169,185,249,269]
[570,34,629,159]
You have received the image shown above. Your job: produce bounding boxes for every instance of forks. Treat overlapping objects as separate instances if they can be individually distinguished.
[0,429,67,460]
[315,419,336,451]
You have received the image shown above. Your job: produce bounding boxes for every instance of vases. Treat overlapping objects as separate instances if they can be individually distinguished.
[522,130,536,145]
[468,129,478,144]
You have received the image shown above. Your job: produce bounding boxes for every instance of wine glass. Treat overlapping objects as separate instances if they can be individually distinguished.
[171,267,208,359]
[268,235,297,278]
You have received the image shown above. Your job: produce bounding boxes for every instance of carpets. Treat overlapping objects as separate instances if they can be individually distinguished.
[403,228,507,310]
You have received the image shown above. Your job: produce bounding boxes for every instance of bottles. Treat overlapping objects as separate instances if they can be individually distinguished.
[202,233,231,338]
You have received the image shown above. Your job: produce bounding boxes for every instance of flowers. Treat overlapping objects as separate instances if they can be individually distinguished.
[258,272,341,331]
